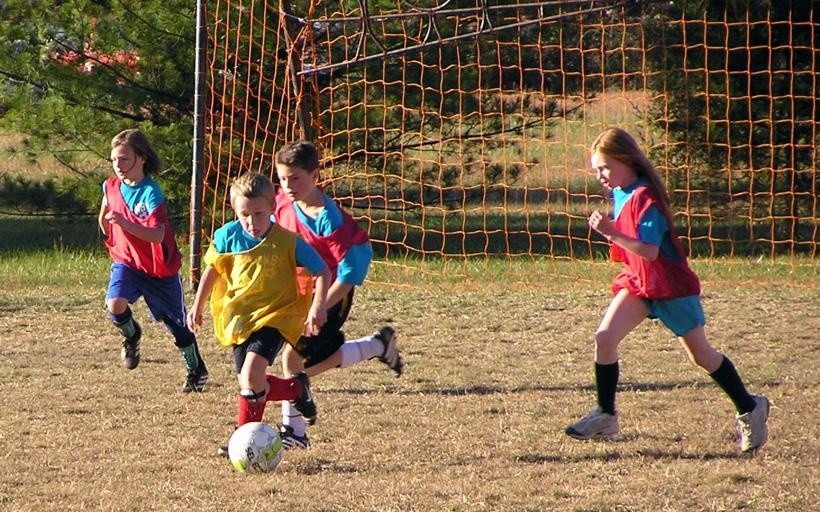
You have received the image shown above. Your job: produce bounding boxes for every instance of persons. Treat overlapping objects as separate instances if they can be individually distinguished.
[96,125,208,394]
[185,169,319,459]
[267,138,405,453]
[562,124,772,455]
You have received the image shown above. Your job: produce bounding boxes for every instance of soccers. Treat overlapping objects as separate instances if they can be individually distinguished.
[228,421,283,474]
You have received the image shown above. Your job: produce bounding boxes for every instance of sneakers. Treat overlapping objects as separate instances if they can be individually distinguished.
[376,325,403,379]
[120,322,142,370]
[274,424,312,452]
[290,370,318,426]
[735,393,770,454]
[565,407,620,439]
[181,364,207,392]
